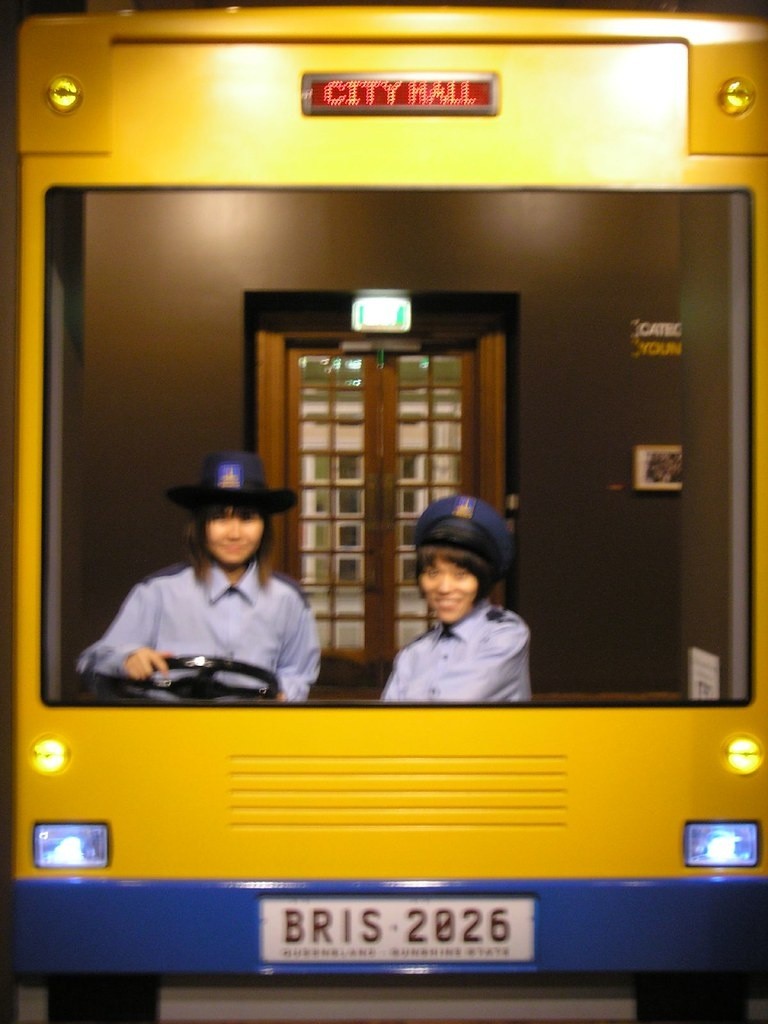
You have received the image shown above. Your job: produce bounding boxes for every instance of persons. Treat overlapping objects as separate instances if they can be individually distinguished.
[379,495,531,704]
[75,454,322,703]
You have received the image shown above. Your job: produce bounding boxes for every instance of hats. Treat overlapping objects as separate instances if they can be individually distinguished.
[413,496,511,583]
[164,452,298,515]
